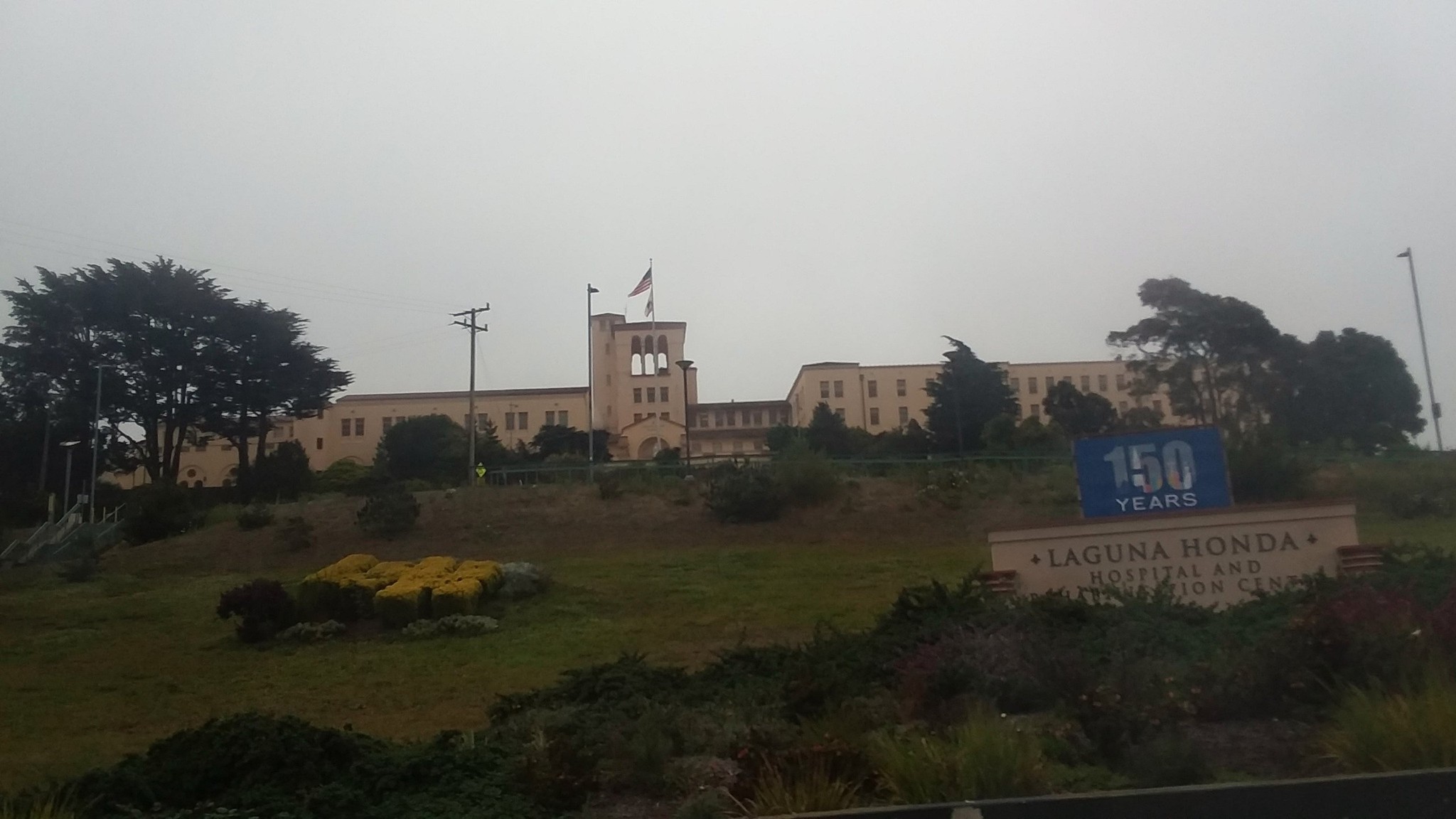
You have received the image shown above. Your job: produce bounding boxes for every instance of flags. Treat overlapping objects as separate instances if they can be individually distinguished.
[645,288,653,317]
[628,268,652,296]
